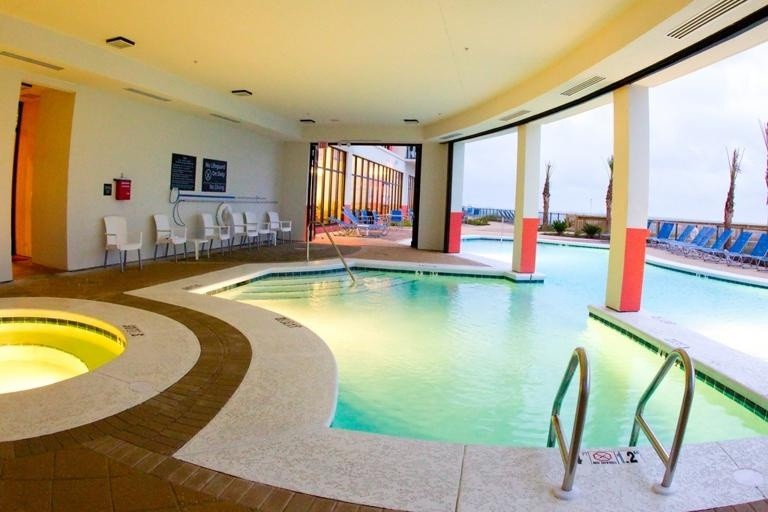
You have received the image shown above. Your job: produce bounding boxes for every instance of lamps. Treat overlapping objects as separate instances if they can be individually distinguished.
[403,118,420,126]
[231,89,253,98]
[299,119,316,125]
[105,36,136,52]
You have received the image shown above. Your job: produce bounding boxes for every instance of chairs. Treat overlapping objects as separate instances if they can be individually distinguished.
[244,211,277,247]
[152,214,188,264]
[324,204,414,238]
[644,220,767,274]
[102,213,144,273]
[199,213,232,256]
[266,211,293,244]
[230,212,258,249]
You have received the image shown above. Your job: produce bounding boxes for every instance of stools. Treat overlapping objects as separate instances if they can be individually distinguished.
[268,230,277,247]
[183,238,211,261]
